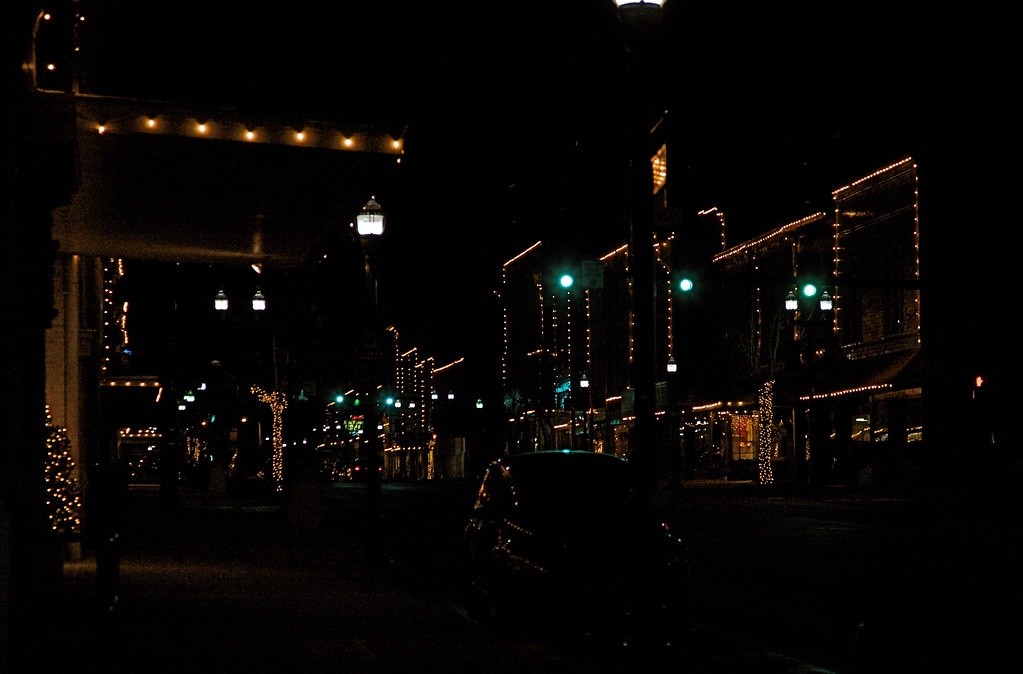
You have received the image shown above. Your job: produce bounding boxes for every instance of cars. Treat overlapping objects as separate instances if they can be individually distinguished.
[463,449,691,614]
